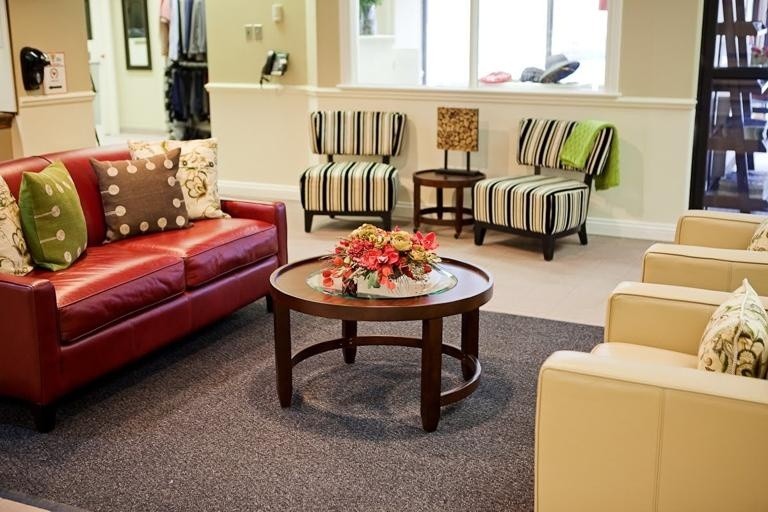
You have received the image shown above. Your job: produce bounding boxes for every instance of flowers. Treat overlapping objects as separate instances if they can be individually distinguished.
[319,223,443,299]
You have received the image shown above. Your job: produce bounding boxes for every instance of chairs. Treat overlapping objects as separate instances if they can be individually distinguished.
[643,210,768,293]
[534,282,768,509]
[473,117,611,261]
[300,109,409,235]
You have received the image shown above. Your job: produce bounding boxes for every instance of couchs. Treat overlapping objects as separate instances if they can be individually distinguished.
[0,142,288,434]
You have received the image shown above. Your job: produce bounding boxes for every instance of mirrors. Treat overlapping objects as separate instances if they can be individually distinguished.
[122,0,152,71]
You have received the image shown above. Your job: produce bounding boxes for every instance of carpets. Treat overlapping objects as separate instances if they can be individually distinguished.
[0,295,604,512]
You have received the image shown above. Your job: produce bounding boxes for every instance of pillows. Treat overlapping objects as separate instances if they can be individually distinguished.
[90,149,194,243]
[696,277,767,379]
[0,156,52,200]
[129,138,233,219]
[39,145,132,246]
[18,157,87,271]
[747,217,768,251]
[0,177,33,277]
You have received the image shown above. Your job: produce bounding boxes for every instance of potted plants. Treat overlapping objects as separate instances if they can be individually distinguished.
[360,1,385,35]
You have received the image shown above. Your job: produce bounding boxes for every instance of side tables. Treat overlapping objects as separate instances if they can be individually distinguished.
[413,170,485,241]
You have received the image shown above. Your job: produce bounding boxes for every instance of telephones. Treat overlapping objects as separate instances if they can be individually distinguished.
[262,50,290,76]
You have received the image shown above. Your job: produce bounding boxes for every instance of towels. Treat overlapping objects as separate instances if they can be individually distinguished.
[561,118,621,190]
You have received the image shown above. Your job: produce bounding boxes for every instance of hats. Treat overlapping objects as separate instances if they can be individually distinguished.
[539,52,580,82]
